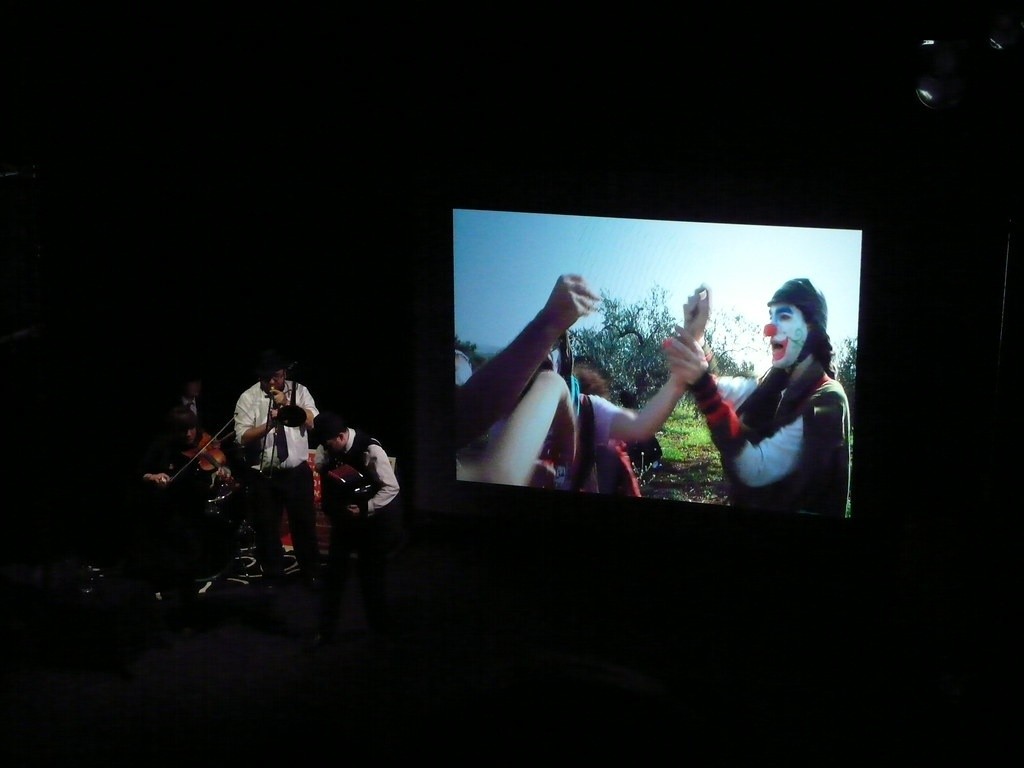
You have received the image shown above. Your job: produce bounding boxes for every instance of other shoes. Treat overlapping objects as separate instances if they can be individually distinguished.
[309,576,321,588]
[264,579,278,588]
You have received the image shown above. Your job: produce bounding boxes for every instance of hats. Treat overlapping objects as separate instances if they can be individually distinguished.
[313,414,347,441]
[257,352,286,374]
[768,277,828,331]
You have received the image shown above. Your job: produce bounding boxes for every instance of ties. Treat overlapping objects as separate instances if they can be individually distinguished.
[273,401,289,464]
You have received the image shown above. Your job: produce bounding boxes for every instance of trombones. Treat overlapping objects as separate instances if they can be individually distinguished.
[258,364,308,478]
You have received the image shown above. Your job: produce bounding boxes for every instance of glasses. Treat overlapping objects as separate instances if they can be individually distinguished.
[259,374,284,384]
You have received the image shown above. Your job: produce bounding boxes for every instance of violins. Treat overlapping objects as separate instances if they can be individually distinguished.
[181,431,229,478]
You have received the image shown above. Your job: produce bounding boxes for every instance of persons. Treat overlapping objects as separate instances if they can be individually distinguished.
[139,412,238,562]
[312,409,401,586]
[488,283,710,496]
[664,278,849,520]
[455,273,602,487]
[165,366,233,445]
[234,354,321,585]
